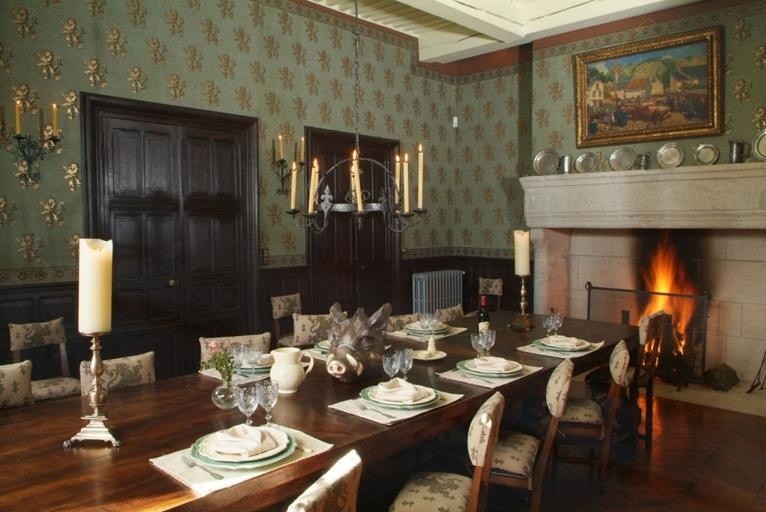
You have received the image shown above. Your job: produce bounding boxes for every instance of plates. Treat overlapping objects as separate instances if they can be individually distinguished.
[457,336,590,378]
[233,346,272,375]
[199,426,289,460]
[404,313,449,340]
[358,384,439,410]
[411,349,446,362]
[370,384,435,404]
[531,133,766,177]
[192,435,297,469]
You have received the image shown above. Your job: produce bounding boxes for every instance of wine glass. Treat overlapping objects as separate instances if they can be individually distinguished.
[237,387,260,426]
[383,354,399,382]
[543,313,563,337]
[395,348,414,378]
[484,330,496,356]
[256,379,285,424]
[471,332,486,356]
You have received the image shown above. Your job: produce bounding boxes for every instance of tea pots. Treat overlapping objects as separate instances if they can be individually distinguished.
[270,346,314,394]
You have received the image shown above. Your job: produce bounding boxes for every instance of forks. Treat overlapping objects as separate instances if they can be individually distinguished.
[183,455,223,480]
[359,404,396,419]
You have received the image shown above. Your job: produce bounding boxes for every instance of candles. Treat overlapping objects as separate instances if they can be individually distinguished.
[514,229,531,275]
[273,132,427,221]
[14,100,21,134]
[76,237,114,334]
[52,103,58,134]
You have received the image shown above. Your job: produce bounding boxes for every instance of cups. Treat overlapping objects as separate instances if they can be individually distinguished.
[478,295,493,334]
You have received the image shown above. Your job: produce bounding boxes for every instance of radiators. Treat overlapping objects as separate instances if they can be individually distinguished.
[413,269,464,314]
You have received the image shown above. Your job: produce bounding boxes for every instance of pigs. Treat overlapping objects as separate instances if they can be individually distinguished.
[325,301,392,384]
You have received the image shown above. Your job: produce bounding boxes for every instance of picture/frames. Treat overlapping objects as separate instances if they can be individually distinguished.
[573,25,726,150]
